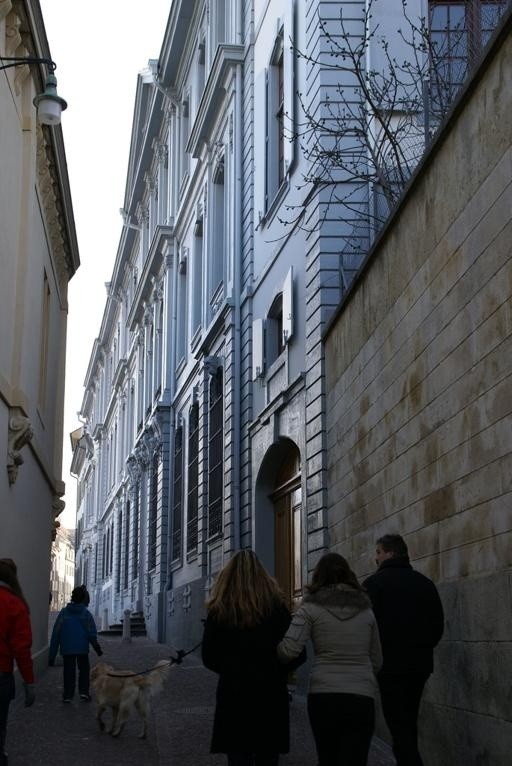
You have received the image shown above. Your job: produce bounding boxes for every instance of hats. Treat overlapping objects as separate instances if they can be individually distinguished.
[71,585,90,606]
[0,558,18,577]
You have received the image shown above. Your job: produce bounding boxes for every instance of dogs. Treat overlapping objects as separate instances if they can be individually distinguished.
[88,658,179,740]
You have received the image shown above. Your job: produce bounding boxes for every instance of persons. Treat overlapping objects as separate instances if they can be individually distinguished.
[1,556,39,766]
[46,583,104,705]
[358,532,446,766]
[272,551,386,764]
[196,549,310,766]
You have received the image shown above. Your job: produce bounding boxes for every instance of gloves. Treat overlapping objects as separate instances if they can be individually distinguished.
[48,660,56,666]
[94,645,104,656]
[22,682,35,708]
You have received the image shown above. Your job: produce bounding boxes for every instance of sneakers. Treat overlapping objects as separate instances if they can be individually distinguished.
[81,694,89,702]
[0,752,10,765]
[63,699,74,705]
